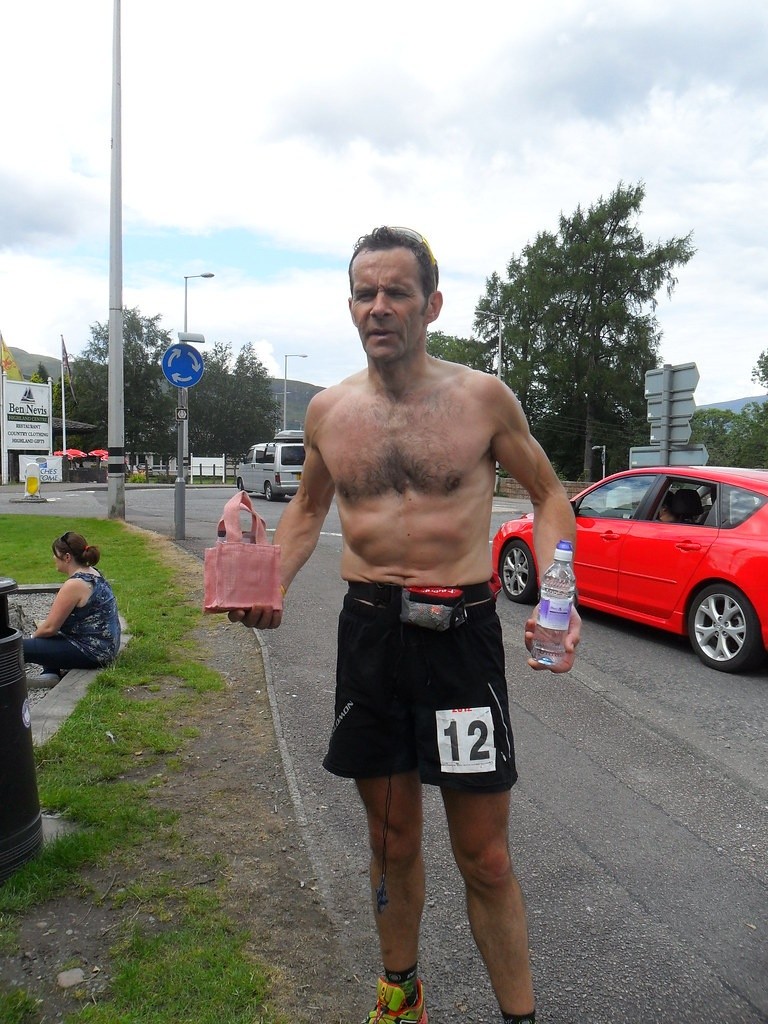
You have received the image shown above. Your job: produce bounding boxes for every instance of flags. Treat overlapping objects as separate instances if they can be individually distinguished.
[0,332,25,381]
[61,335,79,404]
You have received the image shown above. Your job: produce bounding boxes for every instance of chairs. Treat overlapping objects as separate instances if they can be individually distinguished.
[671,488,703,525]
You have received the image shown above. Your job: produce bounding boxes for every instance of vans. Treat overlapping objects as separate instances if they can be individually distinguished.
[236,430,306,501]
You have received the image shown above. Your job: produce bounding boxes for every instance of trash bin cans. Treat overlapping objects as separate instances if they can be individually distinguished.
[1,578,45,886]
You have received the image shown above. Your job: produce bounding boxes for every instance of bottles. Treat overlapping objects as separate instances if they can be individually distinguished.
[530,540,576,666]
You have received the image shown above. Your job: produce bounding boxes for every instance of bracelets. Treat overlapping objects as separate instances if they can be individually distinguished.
[280,583,288,600]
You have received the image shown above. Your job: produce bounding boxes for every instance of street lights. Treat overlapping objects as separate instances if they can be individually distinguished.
[182,272,214,484]
[592,444,606,480]
[474,309,507,495]
[276,353,308,431]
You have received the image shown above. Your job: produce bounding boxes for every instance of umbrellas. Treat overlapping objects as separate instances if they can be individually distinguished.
[65,448,87,458]
[100,454,128,464]
[54,450,73,461]
[89,449,110,456]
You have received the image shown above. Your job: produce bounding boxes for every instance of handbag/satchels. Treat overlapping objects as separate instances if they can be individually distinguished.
[202,489,283,614]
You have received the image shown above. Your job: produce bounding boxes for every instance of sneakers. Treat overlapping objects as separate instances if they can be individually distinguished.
[359,972,429,1024]
[26,673,60,688]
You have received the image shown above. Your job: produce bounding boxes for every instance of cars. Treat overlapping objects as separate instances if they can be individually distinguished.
[137,463,153,475]
[492,465,768,673]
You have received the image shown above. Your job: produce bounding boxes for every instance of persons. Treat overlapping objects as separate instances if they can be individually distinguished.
[19,532,121,680]
[659,489,680,523]
[225,224,584,1023]
